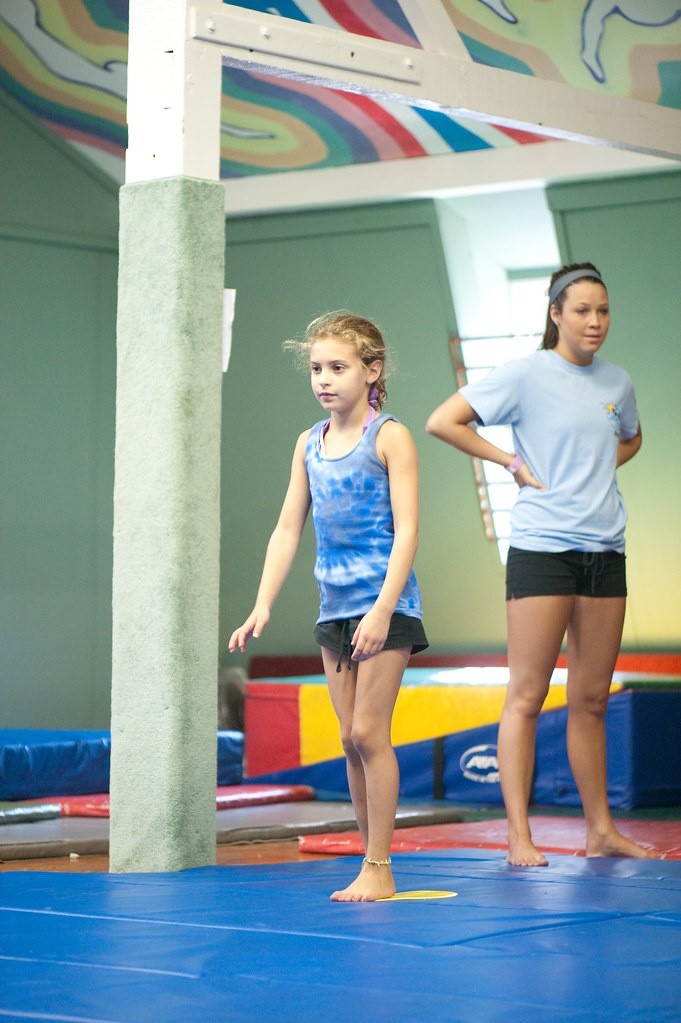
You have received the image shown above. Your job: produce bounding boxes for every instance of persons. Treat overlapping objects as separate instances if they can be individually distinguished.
[425,263,665,868]
[228,313,429,903]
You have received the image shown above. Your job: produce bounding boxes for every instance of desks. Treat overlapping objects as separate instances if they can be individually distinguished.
[241,644,681,810]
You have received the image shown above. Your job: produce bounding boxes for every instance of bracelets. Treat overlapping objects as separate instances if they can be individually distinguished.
[505,455,523,473]
